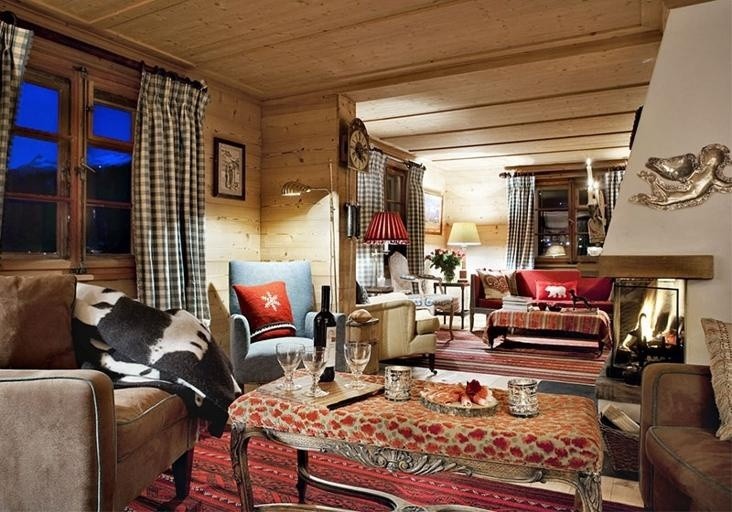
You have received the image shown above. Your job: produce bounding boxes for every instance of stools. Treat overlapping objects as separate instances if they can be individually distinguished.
[537,379,599,416]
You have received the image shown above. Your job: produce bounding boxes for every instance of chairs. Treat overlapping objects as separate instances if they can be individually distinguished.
[0,275,198,512]
[388,251,459,341]
[638,362,732,512]
[355,291,440,371]
[227,259,347,399]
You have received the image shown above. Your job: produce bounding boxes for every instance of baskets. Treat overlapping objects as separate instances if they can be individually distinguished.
[598,416,641,474]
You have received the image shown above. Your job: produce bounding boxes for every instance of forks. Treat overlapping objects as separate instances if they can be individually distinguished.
[326,387,385,411]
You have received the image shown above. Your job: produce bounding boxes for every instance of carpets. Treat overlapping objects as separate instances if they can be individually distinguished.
[125,420,648,512]
[379,328,611,387]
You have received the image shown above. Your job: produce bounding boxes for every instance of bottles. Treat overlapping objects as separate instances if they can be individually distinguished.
[314,285,336,382]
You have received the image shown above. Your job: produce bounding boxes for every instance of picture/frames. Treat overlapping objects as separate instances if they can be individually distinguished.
[213,136,246,201]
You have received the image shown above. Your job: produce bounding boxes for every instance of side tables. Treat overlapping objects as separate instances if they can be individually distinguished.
[433,282,471,329]
[365,286,393,296]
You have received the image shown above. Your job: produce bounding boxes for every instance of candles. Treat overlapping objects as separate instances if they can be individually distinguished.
[584,157,601,205]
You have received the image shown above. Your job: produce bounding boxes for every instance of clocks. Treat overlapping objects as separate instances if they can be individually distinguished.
[339,118,371,174]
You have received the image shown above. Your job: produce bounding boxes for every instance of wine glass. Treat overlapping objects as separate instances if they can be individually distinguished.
[276,343,304,391]
[301,346,329,397]
[343,343,371,390]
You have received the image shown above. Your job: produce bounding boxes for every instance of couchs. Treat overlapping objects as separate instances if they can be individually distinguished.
[469,269,615,332]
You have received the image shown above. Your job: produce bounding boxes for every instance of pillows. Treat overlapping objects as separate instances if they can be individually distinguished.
[475,268,519,300]
[535,279,579,301]
[700,318,732,441]
[232,280,296,343]
[355,280,371,305]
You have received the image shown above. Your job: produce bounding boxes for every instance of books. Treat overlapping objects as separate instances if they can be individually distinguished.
[502,294,533,312]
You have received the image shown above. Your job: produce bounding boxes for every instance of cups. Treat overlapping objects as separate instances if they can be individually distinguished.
[384,365,412,402]
[507,378,539,418]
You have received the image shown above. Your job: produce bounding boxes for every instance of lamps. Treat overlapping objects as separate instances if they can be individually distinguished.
[362,211,411,258]
[545,245,567,258]
[281,157,342,313]
[446,222,481,283]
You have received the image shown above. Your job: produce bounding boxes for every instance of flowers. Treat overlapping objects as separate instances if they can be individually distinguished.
[424,248,466,283]
